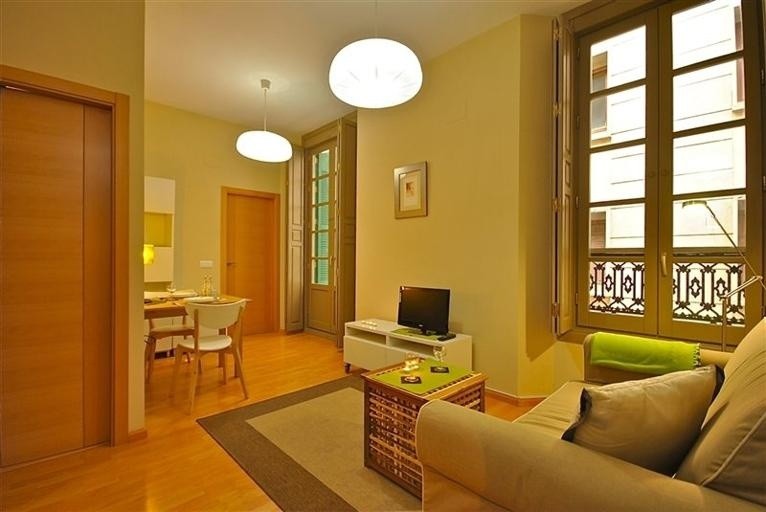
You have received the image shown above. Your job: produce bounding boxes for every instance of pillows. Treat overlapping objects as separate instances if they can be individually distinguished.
[562,365,726,480]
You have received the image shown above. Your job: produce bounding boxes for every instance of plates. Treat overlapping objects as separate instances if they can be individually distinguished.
[184,296,215,304]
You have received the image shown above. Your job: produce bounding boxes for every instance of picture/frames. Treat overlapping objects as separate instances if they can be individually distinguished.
[392,160,428,220]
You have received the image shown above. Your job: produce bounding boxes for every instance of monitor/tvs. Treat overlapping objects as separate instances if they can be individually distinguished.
[397,286,450,335]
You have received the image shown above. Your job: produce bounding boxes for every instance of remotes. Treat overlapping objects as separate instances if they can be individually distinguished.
[438,332,456,341]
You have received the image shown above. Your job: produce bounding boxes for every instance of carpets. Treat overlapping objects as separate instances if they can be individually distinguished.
[197,370,425,512]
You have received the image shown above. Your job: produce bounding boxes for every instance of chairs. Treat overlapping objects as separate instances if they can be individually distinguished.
[144,286,249,400]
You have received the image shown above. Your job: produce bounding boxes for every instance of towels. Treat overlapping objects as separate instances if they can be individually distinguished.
[589,332,701,373]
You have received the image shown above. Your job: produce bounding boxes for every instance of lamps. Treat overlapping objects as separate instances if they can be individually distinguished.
[236,3,425,164]
[144,244,156,265]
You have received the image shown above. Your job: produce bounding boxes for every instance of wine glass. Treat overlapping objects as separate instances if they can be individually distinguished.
[433,344,447,371]
[403,350,419,383]
[166,282,176,303]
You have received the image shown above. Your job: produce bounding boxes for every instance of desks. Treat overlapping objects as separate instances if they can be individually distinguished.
[341,319,472,377]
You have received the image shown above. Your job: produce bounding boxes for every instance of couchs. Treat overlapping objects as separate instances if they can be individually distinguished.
[413,315,766,510]
